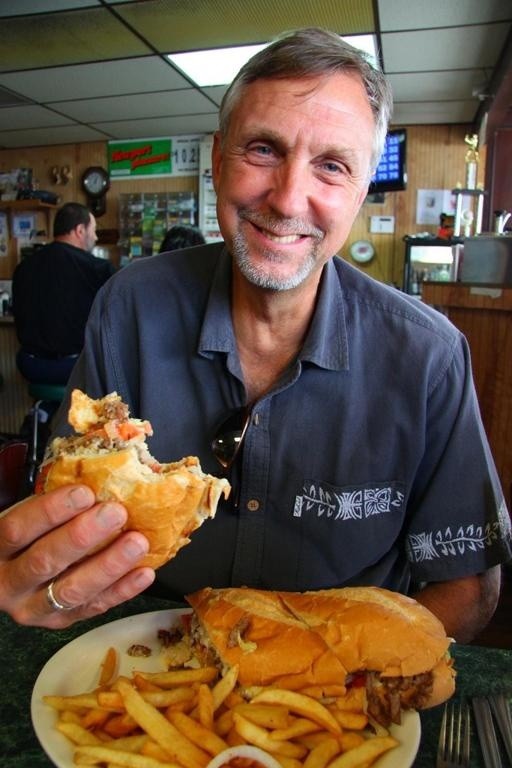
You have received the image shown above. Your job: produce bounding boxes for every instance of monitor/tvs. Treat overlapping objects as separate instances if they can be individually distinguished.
[365,127,407,194]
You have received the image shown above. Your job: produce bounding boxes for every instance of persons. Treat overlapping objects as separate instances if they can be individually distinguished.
[0,27,510,646]
[12,199,121,402]
[157,225,210,256]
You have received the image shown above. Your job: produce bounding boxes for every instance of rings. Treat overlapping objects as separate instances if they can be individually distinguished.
[44,582,71,613]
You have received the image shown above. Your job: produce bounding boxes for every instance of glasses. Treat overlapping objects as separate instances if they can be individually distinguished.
[205,406,253,514]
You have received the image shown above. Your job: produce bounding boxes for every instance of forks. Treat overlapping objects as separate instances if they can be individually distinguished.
[436,704,472,768]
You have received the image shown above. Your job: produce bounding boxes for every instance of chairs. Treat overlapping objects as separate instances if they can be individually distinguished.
[14,351,83,483]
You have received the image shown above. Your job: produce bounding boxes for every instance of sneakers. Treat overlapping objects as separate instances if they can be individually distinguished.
[19,415,48,445]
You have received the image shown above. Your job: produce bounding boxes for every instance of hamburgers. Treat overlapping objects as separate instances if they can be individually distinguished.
[36,391,232,574]
[183,586,456,725]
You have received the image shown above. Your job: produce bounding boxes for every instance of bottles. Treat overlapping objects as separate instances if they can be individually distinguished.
[465,146,477,188]
[410,268,430,298]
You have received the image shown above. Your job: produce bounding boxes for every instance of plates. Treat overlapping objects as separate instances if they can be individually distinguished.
[32,608,422,768]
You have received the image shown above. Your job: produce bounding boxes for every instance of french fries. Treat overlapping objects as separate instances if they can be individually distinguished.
[40,647,400,766]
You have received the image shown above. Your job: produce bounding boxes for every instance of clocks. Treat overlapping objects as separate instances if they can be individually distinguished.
[78,165,112,219]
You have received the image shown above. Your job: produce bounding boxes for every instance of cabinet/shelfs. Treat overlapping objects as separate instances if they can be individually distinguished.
[415,277,511,651]
